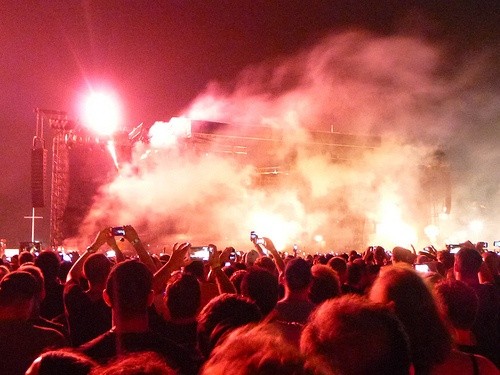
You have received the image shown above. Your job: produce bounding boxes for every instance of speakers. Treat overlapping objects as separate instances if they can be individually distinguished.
[31,148,48,207]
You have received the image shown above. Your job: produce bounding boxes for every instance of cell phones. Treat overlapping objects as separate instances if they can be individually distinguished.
[255,238,265,245]
[188,246,212,261]
[414,264,429,273]
[494,241,500,247]
[251,231,256,239]
[449,244,462,253]
[230,252,241,259]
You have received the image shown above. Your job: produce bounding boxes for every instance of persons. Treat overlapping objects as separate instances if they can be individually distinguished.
[202,321,314,375]
[194,292,265,360]
[366,264,500,375]
[0,270,67,374]
[0,224,500,374]
[299,292,416,375]
[76,260,200,374]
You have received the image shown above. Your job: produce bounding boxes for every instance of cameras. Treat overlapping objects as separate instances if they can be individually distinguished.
[483,242,488,248]
[112,227,126,236]
